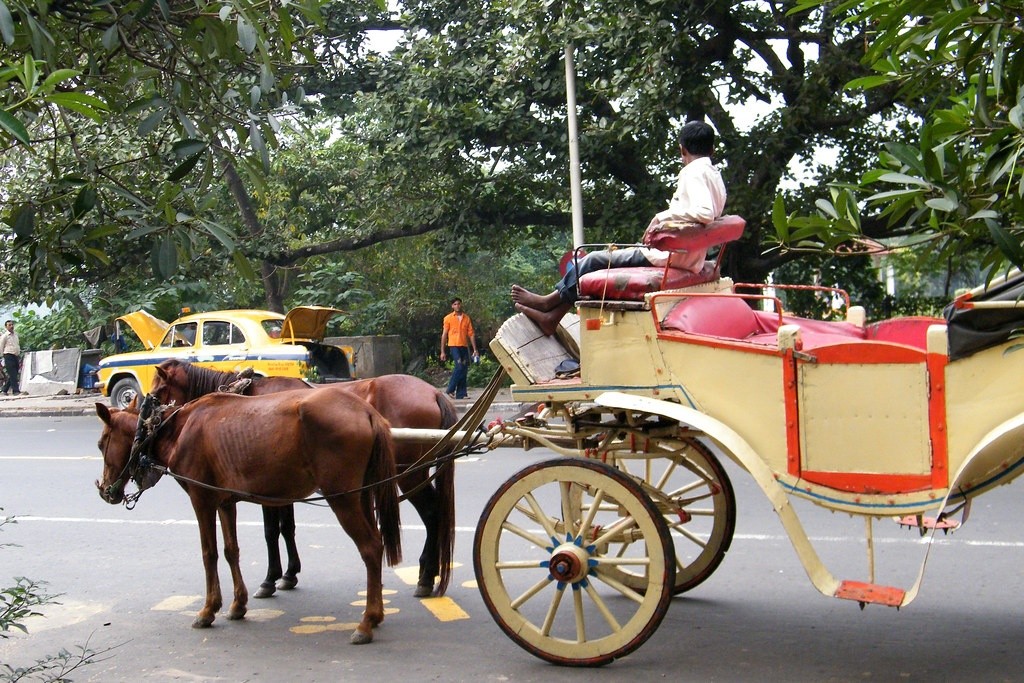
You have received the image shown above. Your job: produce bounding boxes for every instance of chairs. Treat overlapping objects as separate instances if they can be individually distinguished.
[207,326,223,345]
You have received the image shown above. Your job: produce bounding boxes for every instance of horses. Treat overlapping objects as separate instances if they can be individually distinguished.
[151,358,459,599]
[93,388,403,646]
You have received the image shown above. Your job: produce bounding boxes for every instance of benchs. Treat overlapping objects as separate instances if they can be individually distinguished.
[573,215,747,313]
[649,283,866,351]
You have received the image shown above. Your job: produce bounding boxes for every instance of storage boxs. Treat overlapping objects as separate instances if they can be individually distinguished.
[78,374,98,389]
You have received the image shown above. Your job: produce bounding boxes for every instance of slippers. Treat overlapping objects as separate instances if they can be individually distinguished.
[554,359,580,371]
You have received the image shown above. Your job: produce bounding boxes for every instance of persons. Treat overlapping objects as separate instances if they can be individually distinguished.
[511,120,727,337]
[440,297,479,400]
[0,320,23,396]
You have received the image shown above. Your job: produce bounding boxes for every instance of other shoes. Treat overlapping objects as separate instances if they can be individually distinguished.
[0,390,9,396]
[14,392,22,396]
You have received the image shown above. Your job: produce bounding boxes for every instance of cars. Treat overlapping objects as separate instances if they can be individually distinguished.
[94,306,358,411]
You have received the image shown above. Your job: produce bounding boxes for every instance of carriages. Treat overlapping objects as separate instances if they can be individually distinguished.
[96,215,1024,664]
